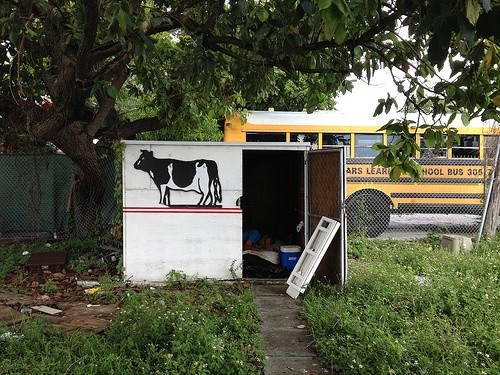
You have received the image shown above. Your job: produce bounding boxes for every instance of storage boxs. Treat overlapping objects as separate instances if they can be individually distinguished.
[280,245,301,269]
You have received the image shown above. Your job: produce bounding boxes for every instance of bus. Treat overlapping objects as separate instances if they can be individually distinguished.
[224,110,500,239]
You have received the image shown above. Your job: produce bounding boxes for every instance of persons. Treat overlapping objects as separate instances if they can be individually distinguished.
[243,229,275,252]
